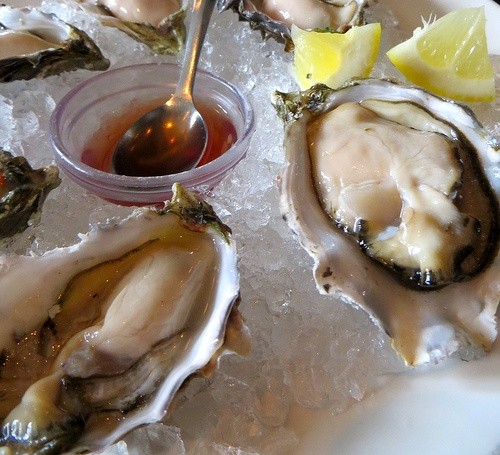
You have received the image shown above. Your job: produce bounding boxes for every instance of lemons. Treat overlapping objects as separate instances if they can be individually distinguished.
[289,4,497,103]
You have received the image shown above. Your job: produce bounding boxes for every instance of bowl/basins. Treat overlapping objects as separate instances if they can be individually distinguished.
[46,63,255,210]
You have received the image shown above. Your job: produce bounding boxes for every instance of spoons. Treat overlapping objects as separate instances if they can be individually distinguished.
[108,0,216,181]
